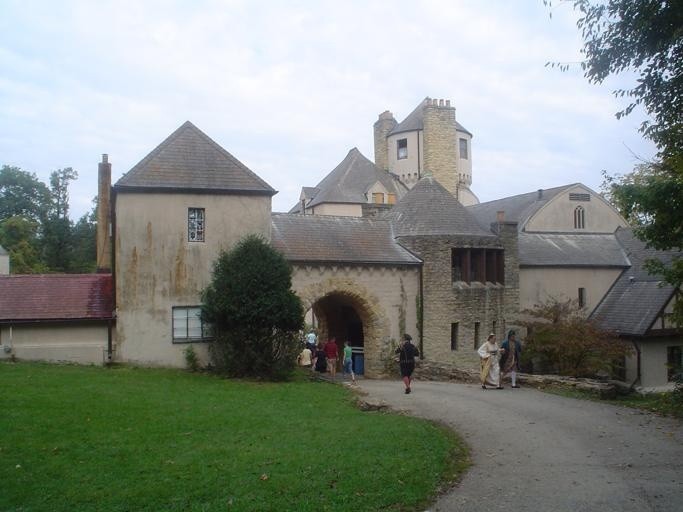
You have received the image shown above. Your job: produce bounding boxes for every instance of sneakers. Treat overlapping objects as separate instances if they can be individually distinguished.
[405,387,412,393]
[481,385,521,390]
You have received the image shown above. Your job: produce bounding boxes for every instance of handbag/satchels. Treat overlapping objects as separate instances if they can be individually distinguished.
[411,346,420,358]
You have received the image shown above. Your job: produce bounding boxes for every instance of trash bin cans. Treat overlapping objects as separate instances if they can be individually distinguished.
[352,347,365,375]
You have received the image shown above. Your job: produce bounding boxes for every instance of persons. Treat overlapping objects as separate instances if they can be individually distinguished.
[476,333,503,390]
[498,329,521,389]
[297,327,356,383]
[395,333,420,393]
